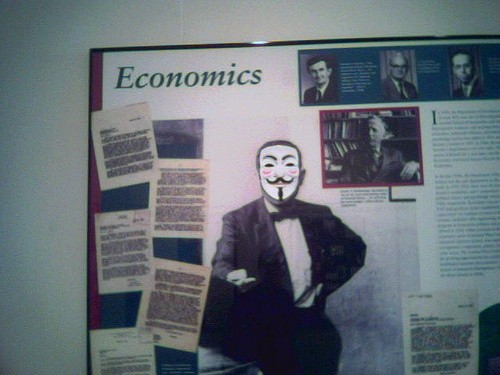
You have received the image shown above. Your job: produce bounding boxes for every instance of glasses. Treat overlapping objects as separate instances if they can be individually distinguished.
[390,64,407,69]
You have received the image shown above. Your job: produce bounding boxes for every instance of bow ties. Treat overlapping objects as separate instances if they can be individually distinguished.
[270,204,298,222]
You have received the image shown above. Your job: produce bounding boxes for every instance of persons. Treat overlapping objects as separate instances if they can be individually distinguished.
[449,50,488,98]
[378,49,420,100]
[338,113,421,185]
[303,55,336,101]
[193,138,369,375]
